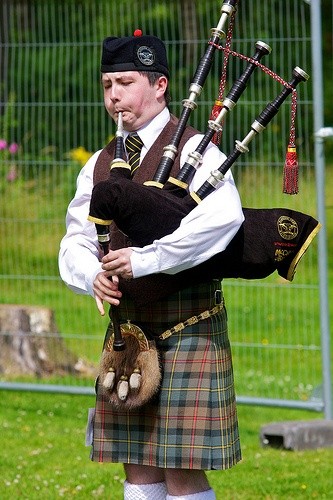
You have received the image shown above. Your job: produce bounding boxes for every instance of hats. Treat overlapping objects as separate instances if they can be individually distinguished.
[100,29,169,81]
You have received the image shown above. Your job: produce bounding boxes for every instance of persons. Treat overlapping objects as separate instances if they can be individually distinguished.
[58,30,245,500]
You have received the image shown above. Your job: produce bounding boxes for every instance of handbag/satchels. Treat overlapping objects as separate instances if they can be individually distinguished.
[99,321,164,408]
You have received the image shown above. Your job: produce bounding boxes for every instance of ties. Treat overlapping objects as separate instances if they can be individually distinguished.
[124,135,143,178]
